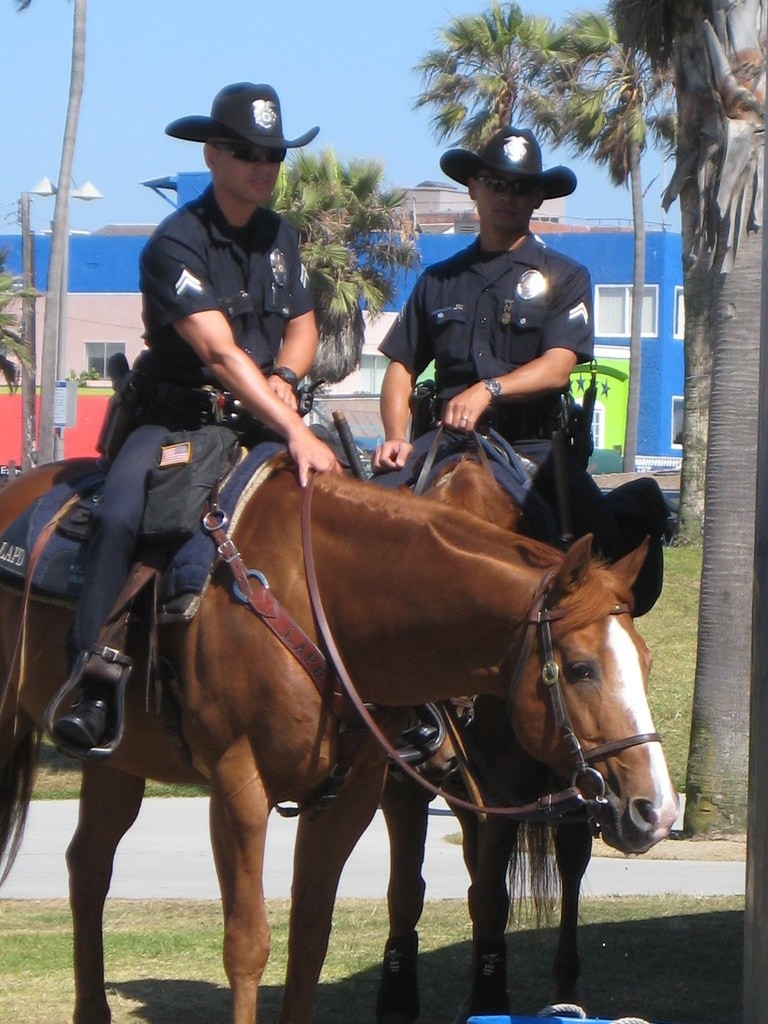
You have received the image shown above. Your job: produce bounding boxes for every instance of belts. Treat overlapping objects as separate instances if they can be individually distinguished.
[435,396,567,434]
[139,377,235,415]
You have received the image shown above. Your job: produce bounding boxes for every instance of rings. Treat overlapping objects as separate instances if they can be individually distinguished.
[460,416,467,421]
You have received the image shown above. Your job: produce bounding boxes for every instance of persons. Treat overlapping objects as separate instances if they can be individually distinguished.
[368,126,605,547]
[53,82,437,745]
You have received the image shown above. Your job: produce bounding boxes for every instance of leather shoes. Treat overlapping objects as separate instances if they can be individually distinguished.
[390,723,437,752]
[51,699,115,753]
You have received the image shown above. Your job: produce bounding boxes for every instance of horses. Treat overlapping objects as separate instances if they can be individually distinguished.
[0,425,680,1024]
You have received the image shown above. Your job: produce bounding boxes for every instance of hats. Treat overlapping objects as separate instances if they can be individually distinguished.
[165,83,319,149]
[440,127,577,200]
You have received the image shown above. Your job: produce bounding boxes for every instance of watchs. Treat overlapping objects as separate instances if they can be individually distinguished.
[483,378,501,398]
[269,366,298,386]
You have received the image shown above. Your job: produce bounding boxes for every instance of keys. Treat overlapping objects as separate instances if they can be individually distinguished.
[209,392,225,423]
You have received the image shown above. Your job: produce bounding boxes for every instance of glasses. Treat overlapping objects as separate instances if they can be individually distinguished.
[213,141,287,164]
[470,175,542,196]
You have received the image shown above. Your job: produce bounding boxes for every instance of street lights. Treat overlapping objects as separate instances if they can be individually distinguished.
[27,174,105,463]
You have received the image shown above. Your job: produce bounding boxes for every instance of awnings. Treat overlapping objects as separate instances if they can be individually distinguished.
[138,176,177,210]
